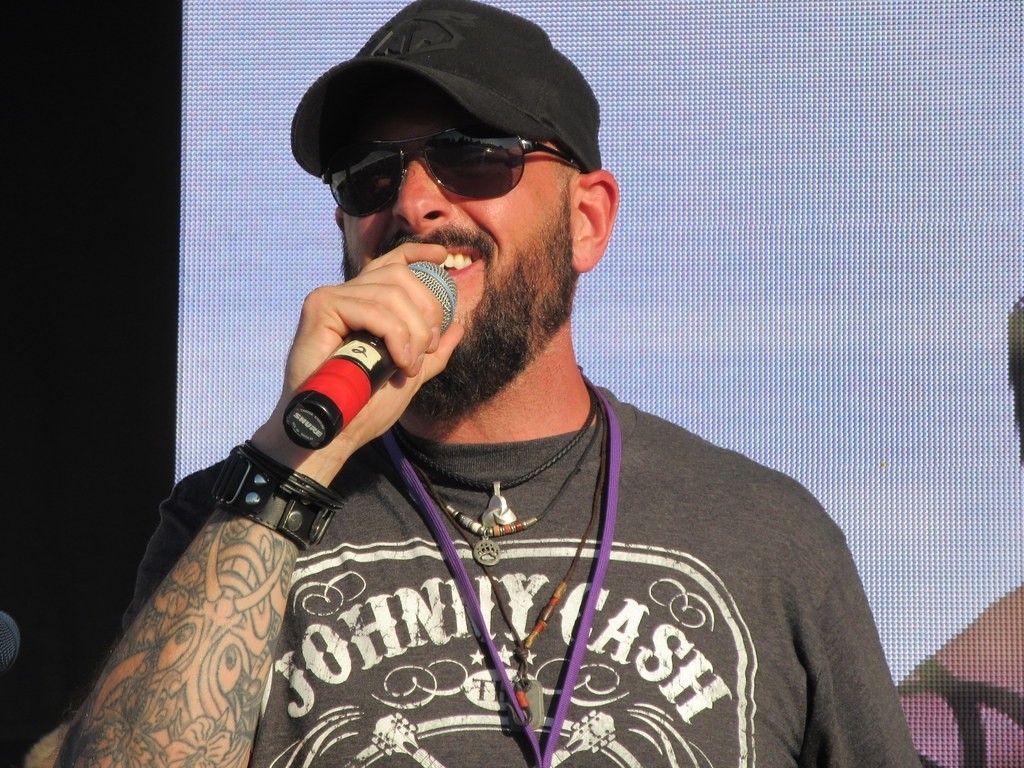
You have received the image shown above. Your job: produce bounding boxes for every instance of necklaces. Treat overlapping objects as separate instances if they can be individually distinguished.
[382,366,623,768]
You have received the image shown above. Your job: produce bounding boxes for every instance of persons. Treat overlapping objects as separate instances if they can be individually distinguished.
[52,0,922,768]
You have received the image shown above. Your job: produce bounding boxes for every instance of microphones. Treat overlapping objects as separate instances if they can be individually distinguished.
[283,261,458,451]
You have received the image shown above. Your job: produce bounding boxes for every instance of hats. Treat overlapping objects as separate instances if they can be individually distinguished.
[290,0,602,168]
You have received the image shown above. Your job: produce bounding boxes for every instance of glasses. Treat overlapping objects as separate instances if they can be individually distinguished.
[322,119,587,218]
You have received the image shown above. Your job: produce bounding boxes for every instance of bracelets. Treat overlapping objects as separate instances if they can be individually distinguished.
[210,441,345,552]
